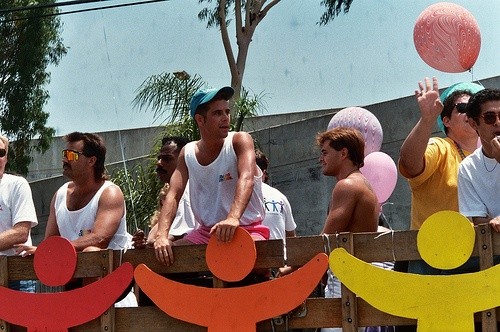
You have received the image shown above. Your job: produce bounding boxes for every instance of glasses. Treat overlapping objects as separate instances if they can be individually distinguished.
[452,101,467,114]
[478,111,500,124]
[0,148,6,157]
[61,149,85,163]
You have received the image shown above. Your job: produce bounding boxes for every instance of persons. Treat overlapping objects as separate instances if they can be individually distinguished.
[456,88,500,232]
[316,129,394,332]
[13,132,135,308]
[399,75,481,275]
[154,86,271,290]
[130,136,297,304]
[0,136,39,293]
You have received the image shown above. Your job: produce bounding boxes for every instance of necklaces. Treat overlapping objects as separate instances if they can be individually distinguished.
[483,156,499,172]
[454,141,466,160]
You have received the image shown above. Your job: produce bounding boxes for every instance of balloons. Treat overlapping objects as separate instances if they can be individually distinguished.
[435,82,485,137]
[414,3,481,72]
[326,106,383,157]
[360,152,398,204]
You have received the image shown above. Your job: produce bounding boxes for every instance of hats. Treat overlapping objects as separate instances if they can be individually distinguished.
[191,86,234,117]
